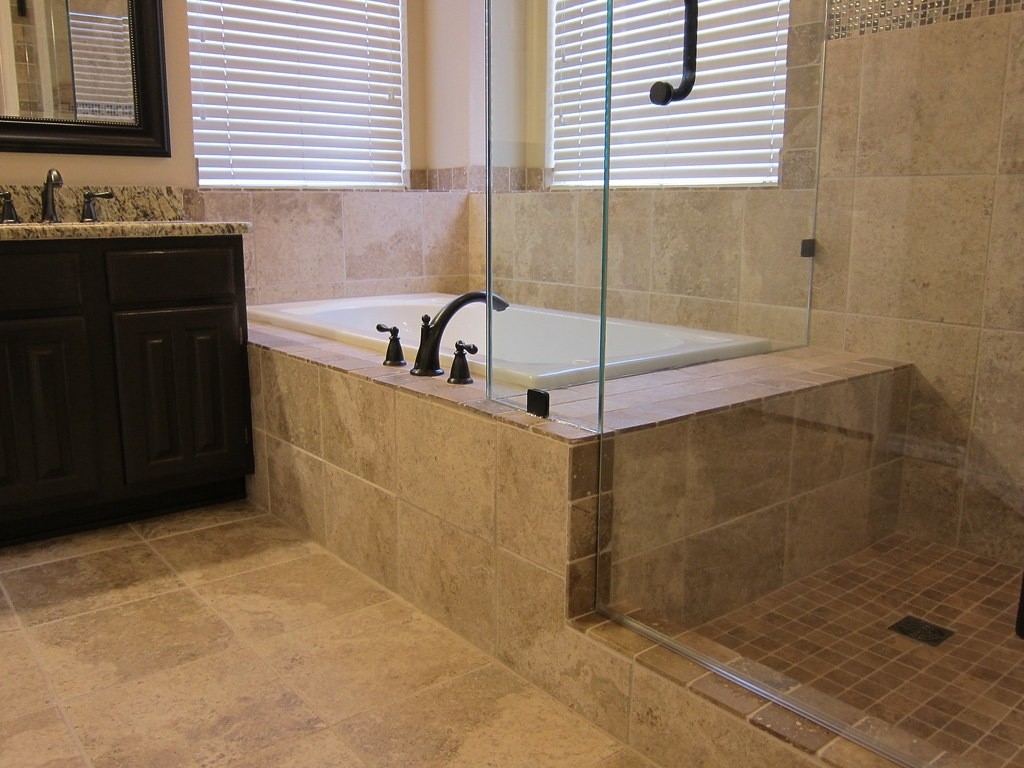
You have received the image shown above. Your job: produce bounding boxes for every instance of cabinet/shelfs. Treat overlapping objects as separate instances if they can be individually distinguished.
[0,219,252,546]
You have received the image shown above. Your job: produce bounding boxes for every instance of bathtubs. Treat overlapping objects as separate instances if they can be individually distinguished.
[244,283,916,697]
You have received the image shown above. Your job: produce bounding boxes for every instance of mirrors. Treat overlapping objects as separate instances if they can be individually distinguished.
[0,0,173,157]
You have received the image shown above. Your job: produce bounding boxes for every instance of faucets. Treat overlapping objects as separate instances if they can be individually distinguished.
[409,289,510,378]
[41,168,65,226]
